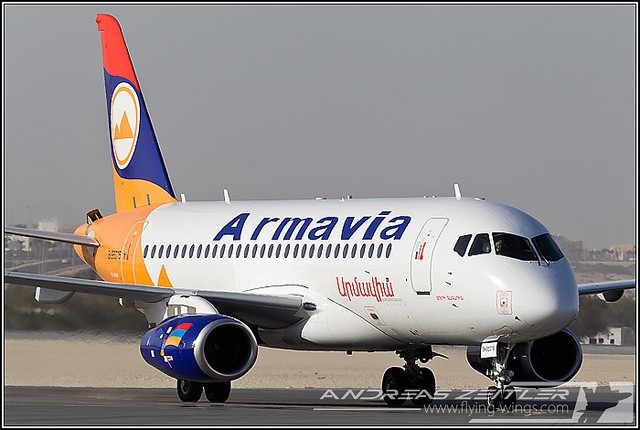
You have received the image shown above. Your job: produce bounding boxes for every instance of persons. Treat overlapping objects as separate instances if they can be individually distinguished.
[495,239,505,255]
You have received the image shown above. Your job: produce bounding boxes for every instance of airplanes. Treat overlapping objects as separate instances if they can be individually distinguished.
[3,14,636,408]
